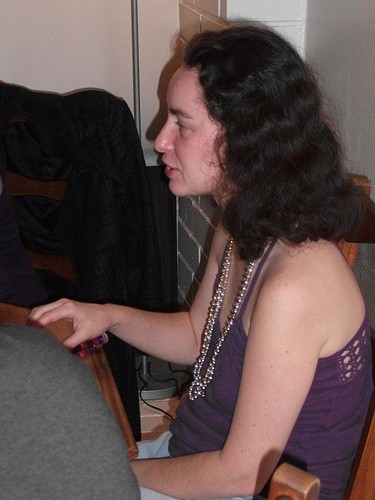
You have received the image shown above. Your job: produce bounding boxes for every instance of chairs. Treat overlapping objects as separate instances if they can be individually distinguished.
[0,81,164,442]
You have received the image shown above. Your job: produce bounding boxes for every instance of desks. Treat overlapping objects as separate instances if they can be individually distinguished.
[0,301,138,462]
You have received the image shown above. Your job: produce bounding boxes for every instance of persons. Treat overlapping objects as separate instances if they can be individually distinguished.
[29,27,374,500]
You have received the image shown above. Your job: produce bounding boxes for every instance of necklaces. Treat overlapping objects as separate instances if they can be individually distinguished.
[189,235,256,401]
